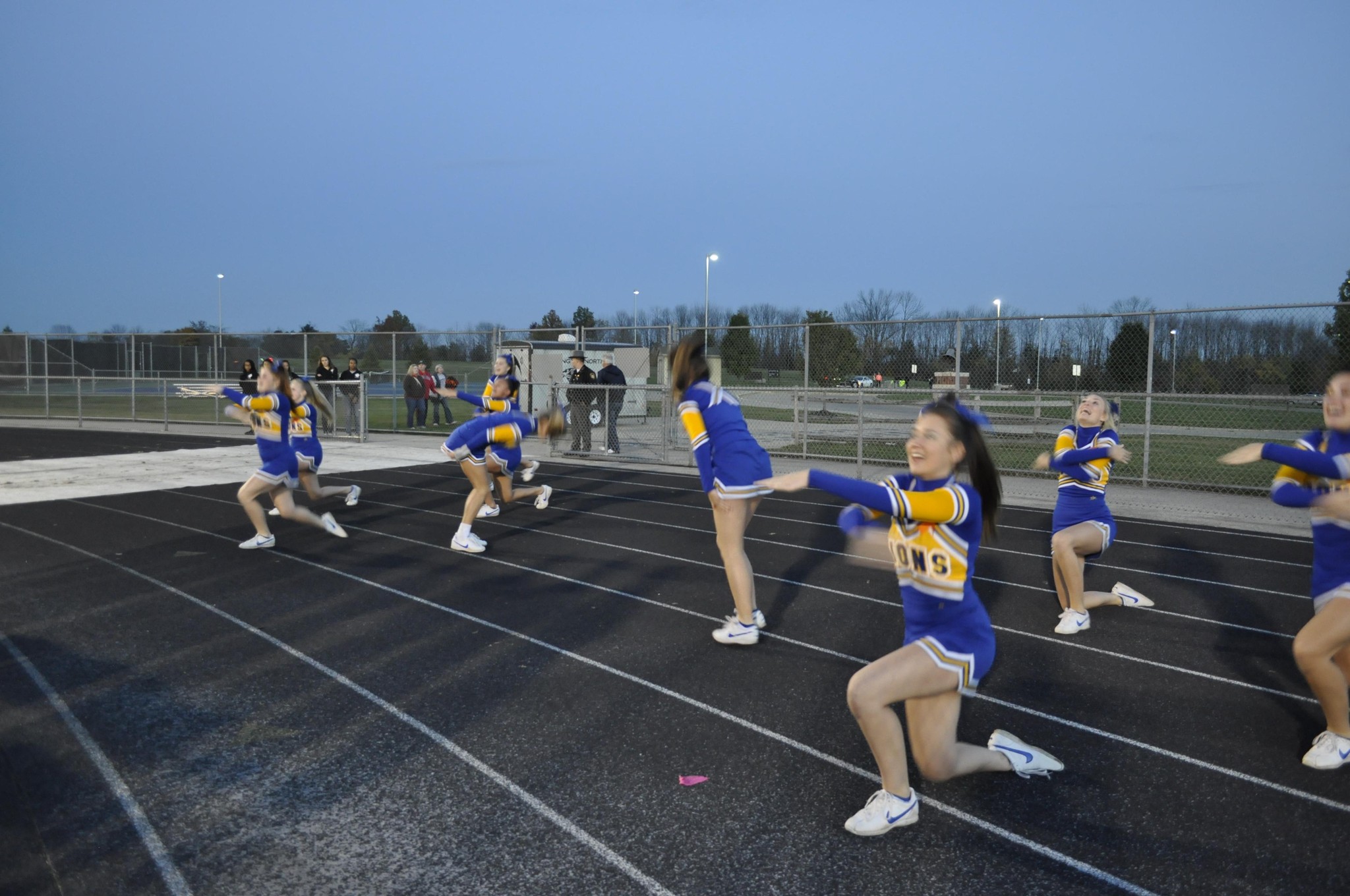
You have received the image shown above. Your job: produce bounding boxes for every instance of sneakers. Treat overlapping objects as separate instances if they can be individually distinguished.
[1110,582,1155,607]
[451,533,486,552]
[476,504,500,518]
[345,485,361,506]
[469,533,488,546]
[1301,730,1350,770]
[490,482,494,490]
[535,485,553,509]
[844,787,919,836]
[323,512,347,538]
[711,615,758,644]
[238,533,275,549]
[987,728,1064,778]
[724,608,766,628]
[269,507,280,515]
[521,461,539,481]
[1054,607,1091,634]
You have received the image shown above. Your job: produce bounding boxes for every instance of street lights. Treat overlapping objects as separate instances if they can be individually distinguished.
[217,274,224,349]
[1170,329,1176,392]
[634,289,639,344]
[705,254,719,353]
[1035,318,1043,392]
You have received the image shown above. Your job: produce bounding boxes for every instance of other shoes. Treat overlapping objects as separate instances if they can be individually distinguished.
[599,446,615,454]
[411,421,457,429]
[245,430,254,435]
[564,449,590,457]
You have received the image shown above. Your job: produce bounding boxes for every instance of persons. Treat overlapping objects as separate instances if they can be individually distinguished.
[1216,369,1350,769]
[595,354,627,454]
[223,377,361,515]
[751,391,1065,836]
[667,330,773,644]
[872,371,934,389]
[565,350,597,457]
[202,361,347,551]
[260,358,274,372]
[404,351,565,553]
[276,360,301,379]
[238,359,259,435]
[315,356,338,436]
[339,358,365,441]
[1035,394,1154,634]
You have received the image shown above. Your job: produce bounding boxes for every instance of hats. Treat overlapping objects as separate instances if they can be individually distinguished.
[568,350,588,359]
[419,360,427,365]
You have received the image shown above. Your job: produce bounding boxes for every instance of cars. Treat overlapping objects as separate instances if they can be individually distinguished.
[852,376,873,389]
[1287,394,1325,407]
[818,371,841,387]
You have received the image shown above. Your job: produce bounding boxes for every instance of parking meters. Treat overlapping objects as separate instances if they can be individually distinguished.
[993,299,1000,384]
